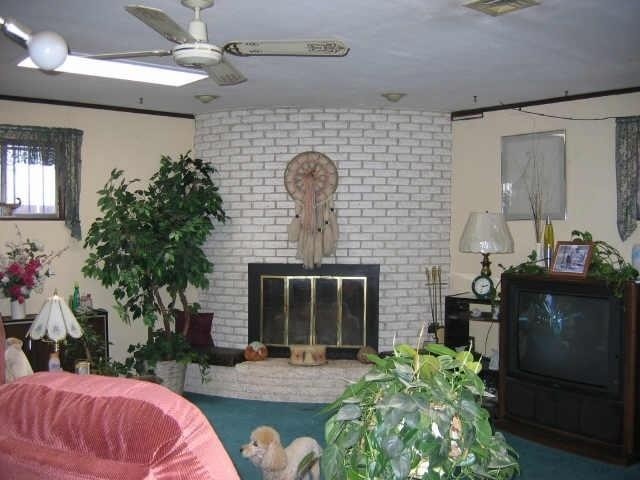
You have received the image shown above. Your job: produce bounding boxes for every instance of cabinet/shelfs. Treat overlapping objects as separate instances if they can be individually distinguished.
[496,271,640,467]
[443,290,500,427]
[4,310,108,372]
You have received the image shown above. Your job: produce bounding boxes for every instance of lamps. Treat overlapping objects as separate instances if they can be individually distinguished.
[459,209,514,275]
[25,288,85,374]
[0,14,68,73]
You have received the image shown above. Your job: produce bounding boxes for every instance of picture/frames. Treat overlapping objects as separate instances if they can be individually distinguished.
[498,128,566,221]
[549,241,594,279]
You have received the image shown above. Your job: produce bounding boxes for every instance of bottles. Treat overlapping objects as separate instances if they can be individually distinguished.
[72,283,93,315]
[543,214,555,271]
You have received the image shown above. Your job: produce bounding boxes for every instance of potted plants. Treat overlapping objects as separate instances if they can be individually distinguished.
[310,323,522,480]
[82,148,232,396]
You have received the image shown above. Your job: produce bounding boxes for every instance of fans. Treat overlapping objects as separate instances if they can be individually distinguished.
[88,0,350,87]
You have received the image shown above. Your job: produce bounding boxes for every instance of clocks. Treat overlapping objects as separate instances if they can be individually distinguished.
[472,276,494,298]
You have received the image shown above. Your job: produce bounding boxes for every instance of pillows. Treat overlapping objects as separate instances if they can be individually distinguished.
[174,308,215,345]
[4,337,33,386]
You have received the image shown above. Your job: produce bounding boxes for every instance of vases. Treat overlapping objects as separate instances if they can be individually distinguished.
[535,242,546,266]
[10,299,26,321]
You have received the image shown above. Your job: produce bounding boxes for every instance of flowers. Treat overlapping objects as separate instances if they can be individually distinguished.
[0,224,71,304]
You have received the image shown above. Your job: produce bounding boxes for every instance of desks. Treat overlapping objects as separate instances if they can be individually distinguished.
[129,374,164,384]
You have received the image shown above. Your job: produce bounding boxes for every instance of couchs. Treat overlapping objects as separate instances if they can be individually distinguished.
[0,371,241,480]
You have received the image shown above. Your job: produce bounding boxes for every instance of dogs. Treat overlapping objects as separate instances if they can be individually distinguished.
[239,425,324,480]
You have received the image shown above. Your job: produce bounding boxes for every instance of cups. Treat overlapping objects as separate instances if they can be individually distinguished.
[74,358,90,375]
